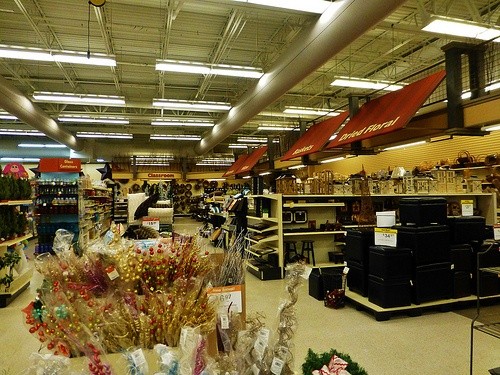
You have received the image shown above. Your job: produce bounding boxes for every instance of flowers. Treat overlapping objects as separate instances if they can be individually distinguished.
[25,235,199,375]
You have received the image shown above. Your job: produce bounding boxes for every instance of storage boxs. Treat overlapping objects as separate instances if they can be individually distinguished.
[203,254,247,348]
[308,198,500,308]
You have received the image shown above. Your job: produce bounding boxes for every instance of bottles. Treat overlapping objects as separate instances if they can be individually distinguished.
[35,177,79,254]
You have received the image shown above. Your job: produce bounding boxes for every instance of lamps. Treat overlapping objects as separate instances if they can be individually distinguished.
[0,0,132,150]
[148,0,500,181]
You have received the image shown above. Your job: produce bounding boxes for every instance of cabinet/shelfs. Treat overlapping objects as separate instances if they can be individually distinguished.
[197,192,248,264]
[115,200,128,224]
[469,240,500,375]
[248,193,497,280]
[0,200,32,308]
[35,179,113,256]
